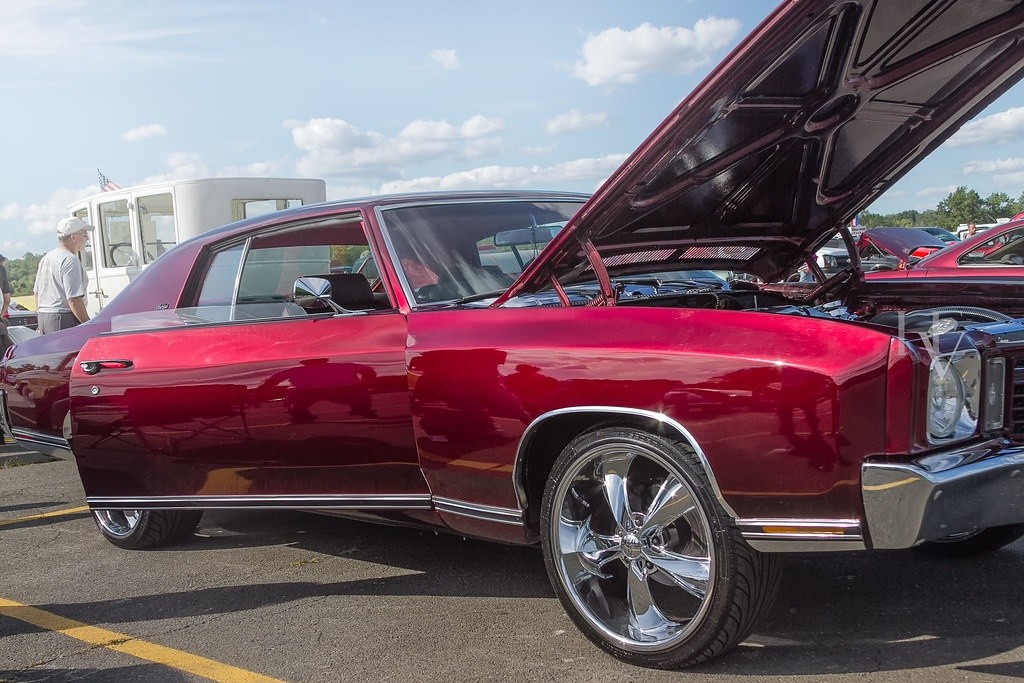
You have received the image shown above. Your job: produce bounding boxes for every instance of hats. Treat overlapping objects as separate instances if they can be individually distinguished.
[56,217,95,236]
[0,254,8,264]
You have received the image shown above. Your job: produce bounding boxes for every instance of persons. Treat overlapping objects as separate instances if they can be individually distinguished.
[797,253,818,282]
[32,218,95,335]
[966,223,976,239]
[0,264,11,444]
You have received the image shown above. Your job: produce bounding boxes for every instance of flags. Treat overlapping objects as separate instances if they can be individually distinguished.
[98,170,123,193]
[850,214,859,226]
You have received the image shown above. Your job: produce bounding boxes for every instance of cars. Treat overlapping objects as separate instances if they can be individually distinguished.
[0,0,1024,669]
